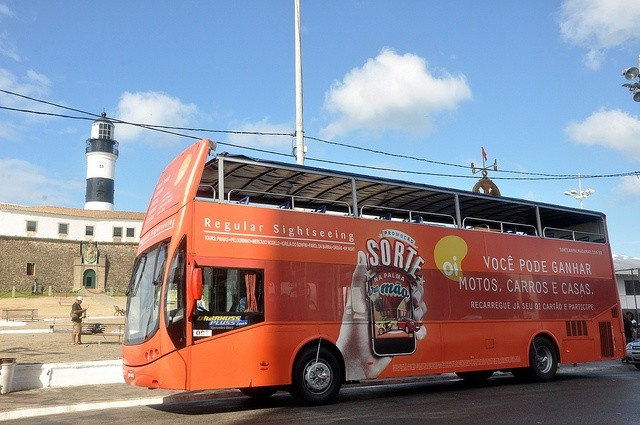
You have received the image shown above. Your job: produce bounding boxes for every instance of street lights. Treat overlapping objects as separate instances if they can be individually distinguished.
[563,170,595,210]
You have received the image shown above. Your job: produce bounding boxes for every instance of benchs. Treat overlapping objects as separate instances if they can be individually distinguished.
[45,315,74,333]
[2,308,41,323]
[81,322,125,345]
[114,305,126,316]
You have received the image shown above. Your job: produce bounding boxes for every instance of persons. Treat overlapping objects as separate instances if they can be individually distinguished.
[69,296,87,345]
[621,311,640,363]
[630,315,637,341]
[333,249,428,381]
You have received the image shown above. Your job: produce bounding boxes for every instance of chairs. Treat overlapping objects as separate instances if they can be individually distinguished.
[280,201,289,209]
[561,235,576,239]
[592,238,605,244]
[577,236,590,241]
[379,212,391,220]
[504,227,516,234]
[522,228,536,236]
[546,233,555,237]
[409,214,421,222]
[314,205,326,213]
[238,196,250,205]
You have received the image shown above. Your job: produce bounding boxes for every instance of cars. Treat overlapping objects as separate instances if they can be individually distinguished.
[626,339,640,370]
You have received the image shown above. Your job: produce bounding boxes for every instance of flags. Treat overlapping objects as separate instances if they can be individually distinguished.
[482,148,487,160]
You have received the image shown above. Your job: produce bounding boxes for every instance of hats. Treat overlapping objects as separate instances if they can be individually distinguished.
[76,296,83,301]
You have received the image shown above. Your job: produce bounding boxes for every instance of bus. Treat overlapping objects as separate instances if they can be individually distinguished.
[120,138,628,408]
